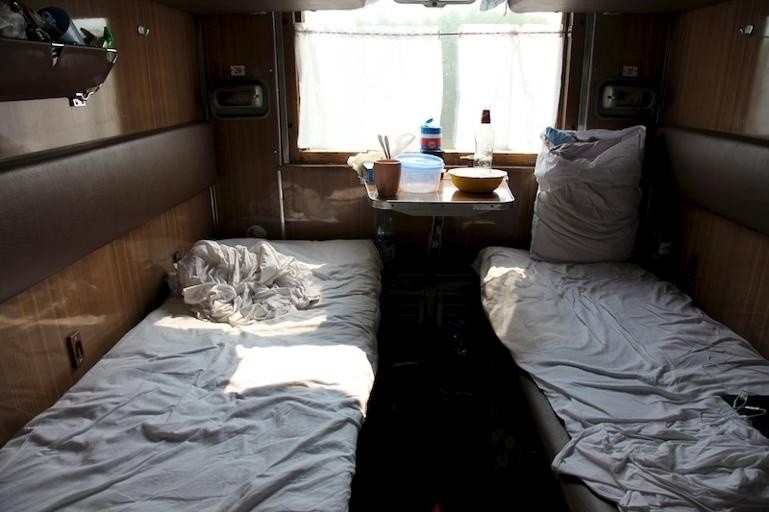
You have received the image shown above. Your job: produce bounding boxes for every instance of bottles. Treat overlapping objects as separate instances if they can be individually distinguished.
[473,108,494,169]
[422,150,445,179]
[421,126,441,150]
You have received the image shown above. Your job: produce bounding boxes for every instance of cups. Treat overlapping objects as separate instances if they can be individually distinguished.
[374,159,402,197]
[34,8,86,47]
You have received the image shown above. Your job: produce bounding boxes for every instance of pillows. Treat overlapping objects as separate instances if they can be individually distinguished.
[528,126,649,264]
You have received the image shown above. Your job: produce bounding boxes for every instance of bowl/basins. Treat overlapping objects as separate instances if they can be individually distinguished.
[449,191,501,203]
[395,154,446,193]
[447,168,506,194]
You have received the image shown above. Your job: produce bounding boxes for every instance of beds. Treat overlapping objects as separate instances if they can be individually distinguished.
[470,246,769,512]
[0,237,384,512]
